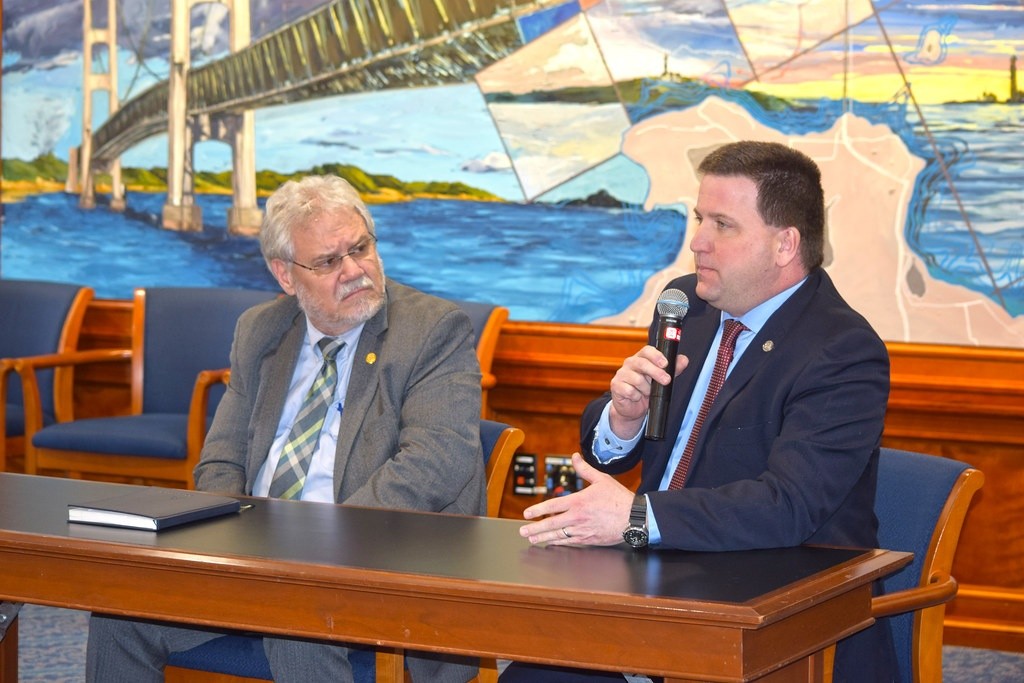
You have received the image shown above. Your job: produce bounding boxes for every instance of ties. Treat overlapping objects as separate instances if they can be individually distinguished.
[667,319,752,491]
[266,337,346,500]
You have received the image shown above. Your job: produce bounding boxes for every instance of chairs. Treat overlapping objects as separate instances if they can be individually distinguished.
[0,279,95,479]
[14,288,285,489]
[166,420,527,683]
[454,299,510,420]
[872,446,984,683]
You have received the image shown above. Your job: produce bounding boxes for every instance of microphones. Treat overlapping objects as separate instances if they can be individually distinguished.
[643,289,689,441]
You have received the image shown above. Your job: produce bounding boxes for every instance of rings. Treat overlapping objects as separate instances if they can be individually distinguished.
[562,527,572,538]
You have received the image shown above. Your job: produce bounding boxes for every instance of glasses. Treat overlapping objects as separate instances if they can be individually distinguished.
[269,231,378,275]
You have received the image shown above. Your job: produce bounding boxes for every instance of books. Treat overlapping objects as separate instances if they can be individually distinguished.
[66,486,256,533]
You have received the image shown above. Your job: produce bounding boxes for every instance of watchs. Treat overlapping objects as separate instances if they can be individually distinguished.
[622,493,649,548]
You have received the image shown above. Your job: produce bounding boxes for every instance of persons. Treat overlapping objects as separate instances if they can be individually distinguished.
[495,141,904,683]
[84,174,486,683]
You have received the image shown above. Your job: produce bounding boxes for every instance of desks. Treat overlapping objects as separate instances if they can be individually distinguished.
[0,468,913,683]
